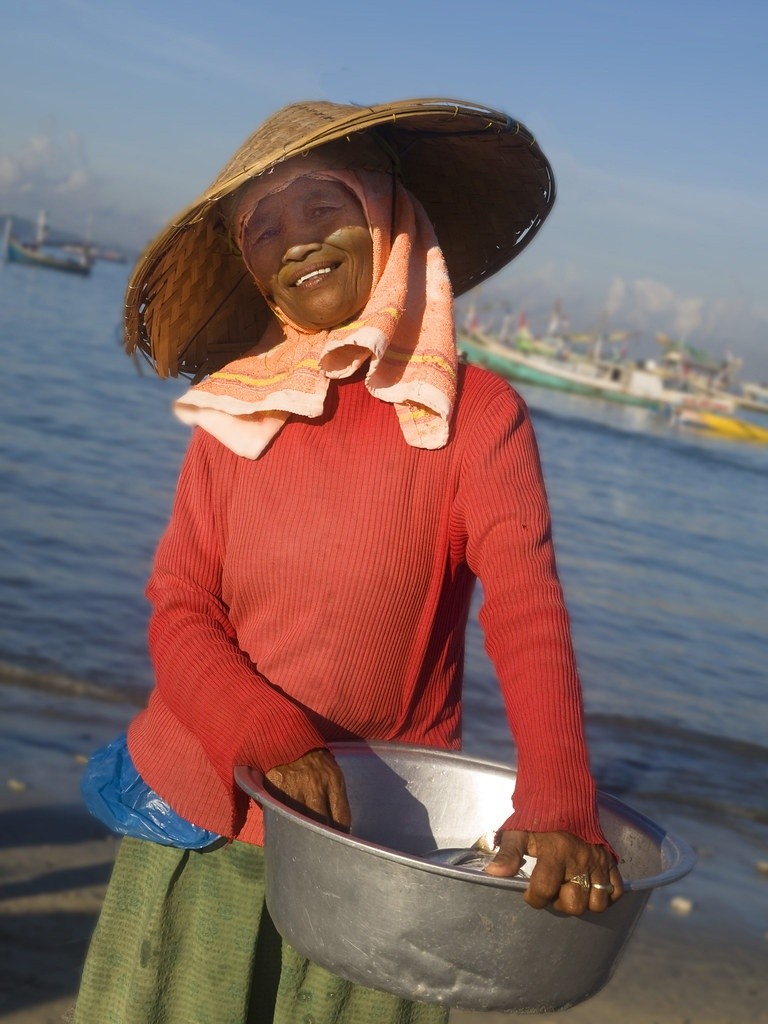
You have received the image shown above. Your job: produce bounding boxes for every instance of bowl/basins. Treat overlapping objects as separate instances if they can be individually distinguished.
[232,741,693,1012]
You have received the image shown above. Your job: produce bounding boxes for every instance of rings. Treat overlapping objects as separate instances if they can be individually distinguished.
[591,884,614,893]
[570,874,591,888]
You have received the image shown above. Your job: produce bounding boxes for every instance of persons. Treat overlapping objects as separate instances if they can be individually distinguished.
[74,100,625,1024]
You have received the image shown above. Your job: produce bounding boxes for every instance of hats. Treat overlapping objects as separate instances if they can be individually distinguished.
[122,100,557,387]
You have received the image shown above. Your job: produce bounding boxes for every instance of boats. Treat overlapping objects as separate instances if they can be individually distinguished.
[7,239,98,276]
[455,298,768,475]
